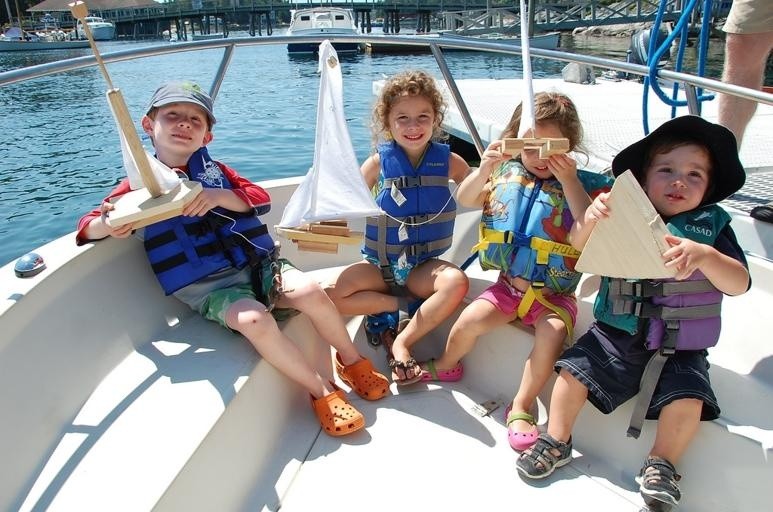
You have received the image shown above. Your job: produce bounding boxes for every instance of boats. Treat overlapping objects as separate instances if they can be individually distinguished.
[284,7,363,55]
[0,34,773,512]
[437,30,562,51]
[1,26,96,51]
[70,17,116,40]
[188,33,224,42]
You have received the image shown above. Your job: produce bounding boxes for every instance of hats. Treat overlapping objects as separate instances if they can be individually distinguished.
[147,81,216,124]
[612,116,745,209]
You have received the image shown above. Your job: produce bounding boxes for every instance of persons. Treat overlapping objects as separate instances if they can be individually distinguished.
[408,91,595,452]
[327,72,482,387]
[77,82,389,436]
[517,116,753,507]
[714,1,773,172]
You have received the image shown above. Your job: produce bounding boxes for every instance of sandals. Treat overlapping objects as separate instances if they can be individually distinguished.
[336,352,390,401]
[309,380,365,436]
[418,359,463,382]
[504,401,538,451]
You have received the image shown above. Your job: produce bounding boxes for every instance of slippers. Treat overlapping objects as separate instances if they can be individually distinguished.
[382,329,423,386]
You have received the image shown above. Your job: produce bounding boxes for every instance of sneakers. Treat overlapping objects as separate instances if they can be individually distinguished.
[516,433,572,479]
[635,455,681,506]
[750,201,773,222]
